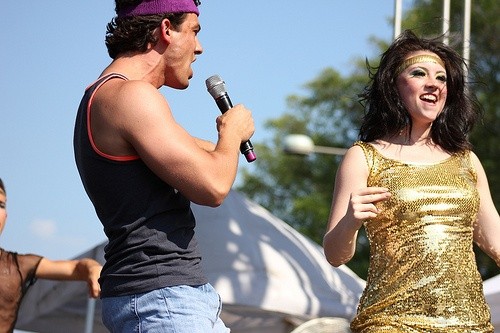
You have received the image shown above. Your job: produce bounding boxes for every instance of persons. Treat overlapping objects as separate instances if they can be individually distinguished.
[0,178,104,333]
[324,28,500,333]
[74,0,254,333]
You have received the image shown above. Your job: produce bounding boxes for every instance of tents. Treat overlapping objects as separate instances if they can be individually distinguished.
[15,188,371,332]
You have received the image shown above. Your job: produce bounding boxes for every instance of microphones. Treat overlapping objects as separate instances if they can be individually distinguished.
[206,74,257,163]
